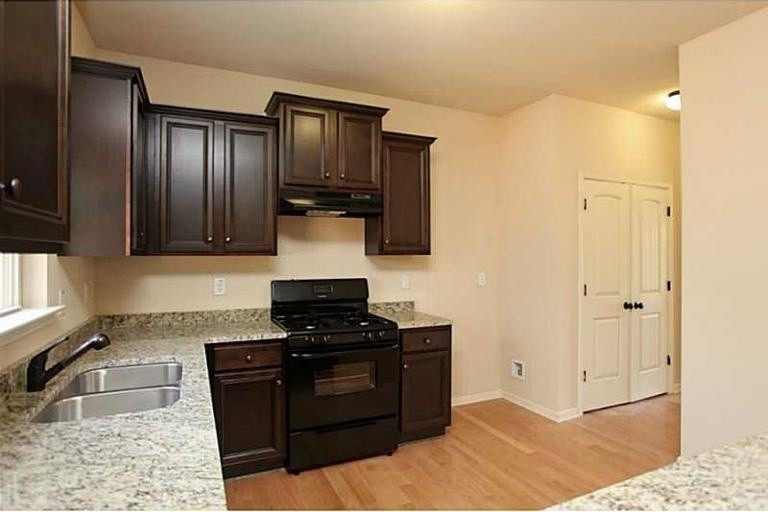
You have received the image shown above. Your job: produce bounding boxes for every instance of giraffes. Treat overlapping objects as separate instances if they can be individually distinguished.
[274,307,401,345]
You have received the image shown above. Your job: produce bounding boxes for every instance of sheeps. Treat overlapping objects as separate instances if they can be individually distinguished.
[26,329,111,393]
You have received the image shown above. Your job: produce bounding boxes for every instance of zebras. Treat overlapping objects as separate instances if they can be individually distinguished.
[286,343,399,473]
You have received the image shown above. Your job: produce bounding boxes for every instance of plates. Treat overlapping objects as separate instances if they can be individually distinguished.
[69,52,146,259]
[264,91,391,197]
[148,101,279,257]
[0,0,65,257]
[206,340,286,481]
[362,128,438,256]
[400,325,451,440]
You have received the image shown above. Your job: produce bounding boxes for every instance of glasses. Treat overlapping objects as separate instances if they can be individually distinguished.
[665,90,680,111]
